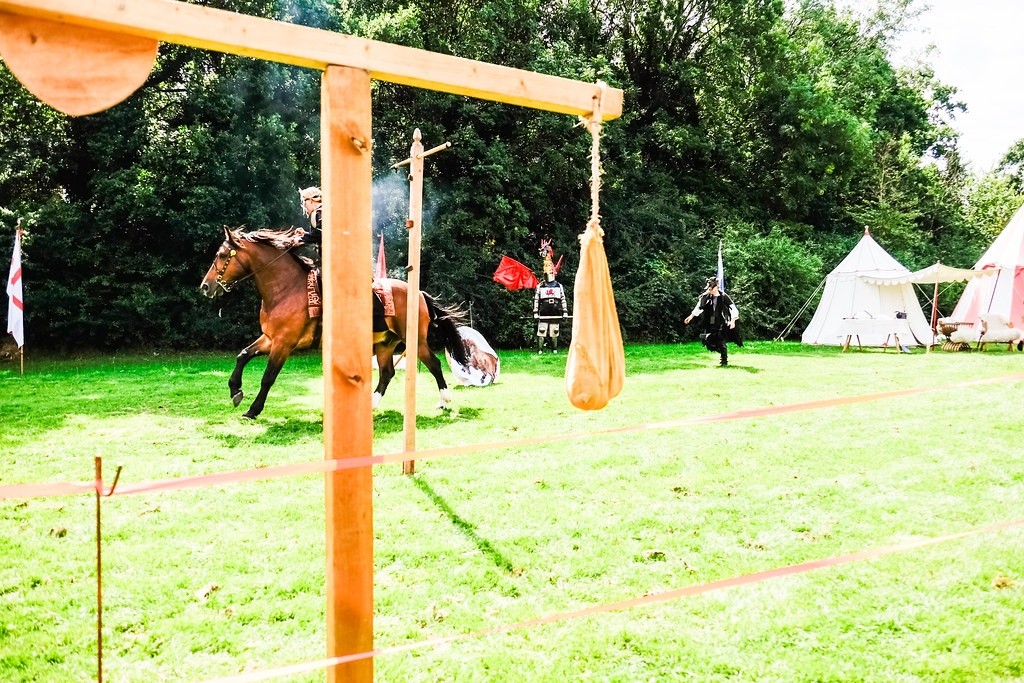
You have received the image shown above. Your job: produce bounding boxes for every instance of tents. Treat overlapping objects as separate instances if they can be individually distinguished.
[801,225,941,347]
[937,202,1024,343]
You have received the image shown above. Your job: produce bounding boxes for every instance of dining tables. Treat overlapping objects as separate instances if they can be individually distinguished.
[837,318,906,354]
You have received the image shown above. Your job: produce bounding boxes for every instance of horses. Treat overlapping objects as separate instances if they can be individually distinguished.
[194,221,470,424]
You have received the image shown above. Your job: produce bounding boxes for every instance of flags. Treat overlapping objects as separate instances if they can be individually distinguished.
[492,255,539,291]
[716,244,724,292]
[375,230,387,278]
[6,225,24,349]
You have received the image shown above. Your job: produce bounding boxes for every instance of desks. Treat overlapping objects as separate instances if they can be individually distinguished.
[940,317,974,353]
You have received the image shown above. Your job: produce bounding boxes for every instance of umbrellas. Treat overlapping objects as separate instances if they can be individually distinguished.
[856,260,1001,353]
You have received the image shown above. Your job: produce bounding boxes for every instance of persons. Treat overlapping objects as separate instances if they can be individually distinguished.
[684,276,744,366]
[533,267,568,355]
[294,186,322,299]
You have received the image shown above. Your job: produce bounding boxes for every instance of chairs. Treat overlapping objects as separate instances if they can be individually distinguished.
[977,313,1022,353]
